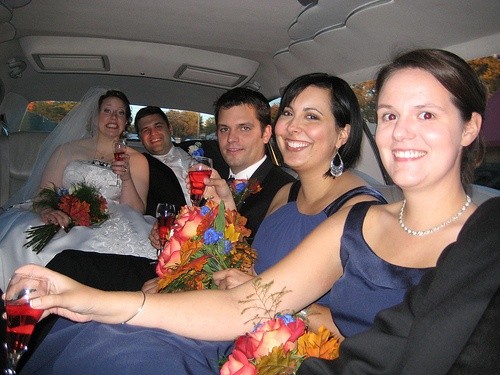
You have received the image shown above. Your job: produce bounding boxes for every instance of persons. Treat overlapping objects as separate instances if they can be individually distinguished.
[0,86,162,301]
[2,48,500,375]
[134,105,207,221]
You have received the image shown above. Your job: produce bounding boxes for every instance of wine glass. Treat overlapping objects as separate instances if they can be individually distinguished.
[188,154,214,209]
[151,202,176,264]
[1,272,50,372]
[110,139,126,190]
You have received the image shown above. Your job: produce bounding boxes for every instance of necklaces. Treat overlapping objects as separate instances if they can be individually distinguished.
[400,194,472,236]
[94,141,115,159]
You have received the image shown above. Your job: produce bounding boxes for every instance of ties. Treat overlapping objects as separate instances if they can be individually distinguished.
[226,177,235,186]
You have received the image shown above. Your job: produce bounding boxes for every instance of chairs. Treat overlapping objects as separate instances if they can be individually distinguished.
[373,184,500,207]
[0,132,50,210]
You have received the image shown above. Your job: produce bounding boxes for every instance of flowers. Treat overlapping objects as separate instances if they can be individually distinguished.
[23,143,342,375]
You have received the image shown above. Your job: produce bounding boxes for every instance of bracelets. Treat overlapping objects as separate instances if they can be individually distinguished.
[119,290,146,324]
